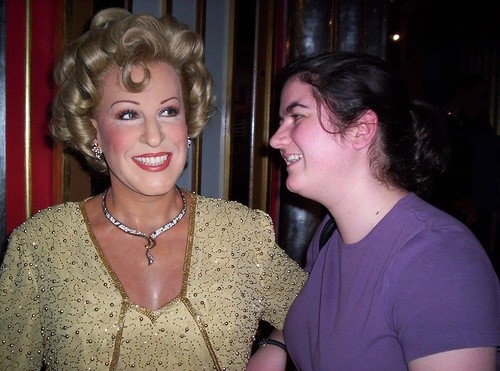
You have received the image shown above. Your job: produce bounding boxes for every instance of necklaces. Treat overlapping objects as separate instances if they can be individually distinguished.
[101,183,186,266]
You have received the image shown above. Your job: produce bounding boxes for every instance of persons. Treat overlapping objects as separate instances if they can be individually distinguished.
[268,49,500,371]
[1,8,310,371]
